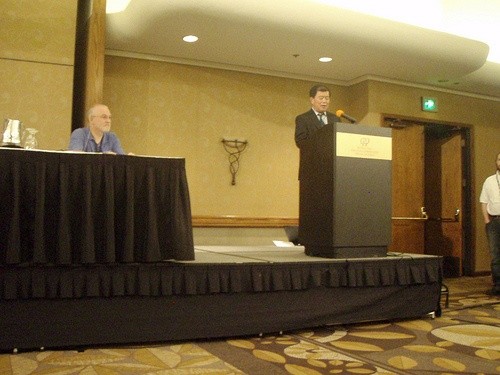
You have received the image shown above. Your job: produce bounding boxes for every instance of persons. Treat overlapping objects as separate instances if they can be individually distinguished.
[480,153,500,297]
[69,104,126,154]
[295,85,343,151]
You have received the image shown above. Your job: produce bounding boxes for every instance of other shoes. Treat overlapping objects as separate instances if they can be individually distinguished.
[491,283,500,294]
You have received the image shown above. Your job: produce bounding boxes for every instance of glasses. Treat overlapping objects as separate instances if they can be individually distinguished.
[315,96,330,102]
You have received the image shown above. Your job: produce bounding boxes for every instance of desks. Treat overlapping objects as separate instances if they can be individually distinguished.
[0,144,196,266]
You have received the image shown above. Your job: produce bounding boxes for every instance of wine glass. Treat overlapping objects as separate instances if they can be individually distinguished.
[24,127,39,149]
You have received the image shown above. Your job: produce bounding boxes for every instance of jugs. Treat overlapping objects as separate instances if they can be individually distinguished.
[2,117,23,147]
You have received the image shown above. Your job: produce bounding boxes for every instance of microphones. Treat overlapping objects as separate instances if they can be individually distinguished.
[335,109,357,124]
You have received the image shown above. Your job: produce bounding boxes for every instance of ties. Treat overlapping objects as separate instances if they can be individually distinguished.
[316,112,325,127]
